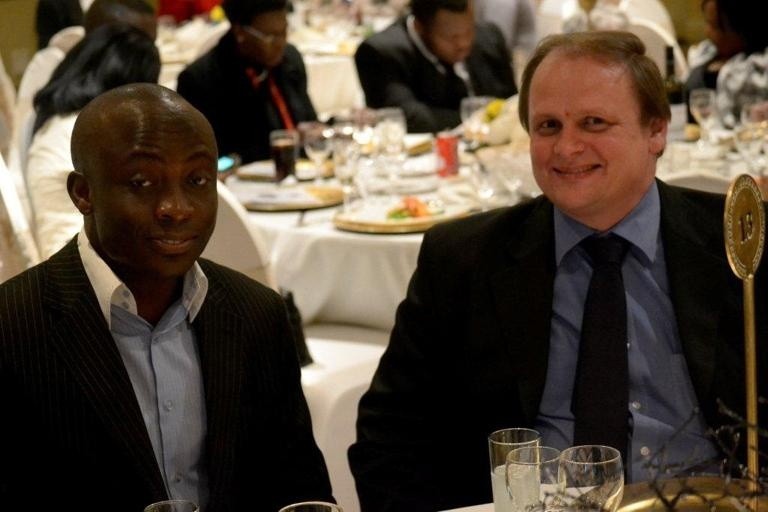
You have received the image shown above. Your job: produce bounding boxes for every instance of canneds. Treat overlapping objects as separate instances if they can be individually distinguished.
[436,131,459,175]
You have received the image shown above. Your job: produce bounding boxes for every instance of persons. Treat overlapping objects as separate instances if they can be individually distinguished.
[0,82,339,512]
[354,0,767,135]
[23,1,335,169]
[347,29,767,512]
[24,18,163,264]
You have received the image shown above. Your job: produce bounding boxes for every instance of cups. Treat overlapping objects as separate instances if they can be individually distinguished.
[503,444,572,510]
[142,499,199,512]
[488,426,546,511]
[559,445,625,511]
[278,500,341,512]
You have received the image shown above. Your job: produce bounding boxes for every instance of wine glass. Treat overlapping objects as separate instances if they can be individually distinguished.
[660,52,766,185]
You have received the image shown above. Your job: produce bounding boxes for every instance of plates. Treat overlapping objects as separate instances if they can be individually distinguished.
[212,96,516,232]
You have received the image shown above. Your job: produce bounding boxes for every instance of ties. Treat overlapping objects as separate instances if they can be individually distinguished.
[570,236,629,484]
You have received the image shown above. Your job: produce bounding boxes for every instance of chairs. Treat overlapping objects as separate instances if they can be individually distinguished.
[0,25,274,289]
[626,18,688,83]
